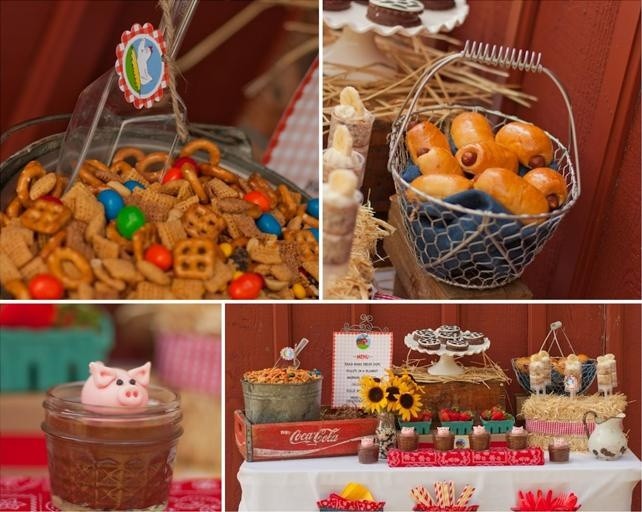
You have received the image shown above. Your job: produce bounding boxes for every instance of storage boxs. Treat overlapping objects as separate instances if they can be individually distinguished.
[234,406,382,464]
[414,370,509,429]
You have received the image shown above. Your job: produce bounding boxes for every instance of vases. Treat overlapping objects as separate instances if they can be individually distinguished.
[372,408,401,462]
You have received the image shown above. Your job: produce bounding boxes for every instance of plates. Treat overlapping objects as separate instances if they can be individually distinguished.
[0,138,277,215]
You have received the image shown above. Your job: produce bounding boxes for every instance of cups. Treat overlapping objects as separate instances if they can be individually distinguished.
[322,104,376,265]
[357,431,571,465]
[40,379,185,510]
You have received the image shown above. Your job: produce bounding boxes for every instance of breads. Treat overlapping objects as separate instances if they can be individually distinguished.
[404,112,567,225]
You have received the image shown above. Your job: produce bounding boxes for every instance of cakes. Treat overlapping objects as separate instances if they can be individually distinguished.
[43,360,182,510]
[358,325,618,464]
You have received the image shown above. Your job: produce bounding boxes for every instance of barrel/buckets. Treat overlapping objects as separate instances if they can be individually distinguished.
[241,366,324,422]
[0,115,319,213]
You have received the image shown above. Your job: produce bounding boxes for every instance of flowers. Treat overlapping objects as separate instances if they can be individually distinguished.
[356,365,428,422]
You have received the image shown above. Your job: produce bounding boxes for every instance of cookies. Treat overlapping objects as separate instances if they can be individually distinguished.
[0,139,319,300]
[323,85,375,280]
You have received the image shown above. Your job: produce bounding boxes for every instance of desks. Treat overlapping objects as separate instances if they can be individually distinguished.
[232,440,641,511]
